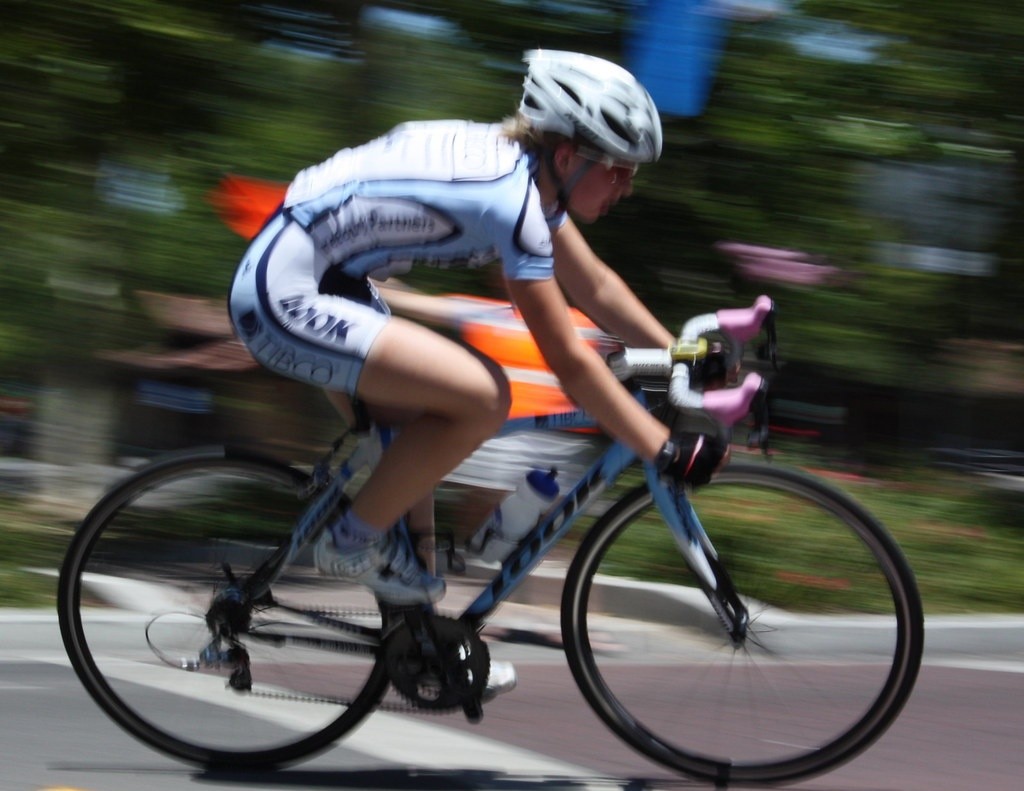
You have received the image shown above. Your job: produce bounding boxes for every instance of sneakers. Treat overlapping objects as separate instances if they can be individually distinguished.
[315,528,446,604]
[423,646,518,700]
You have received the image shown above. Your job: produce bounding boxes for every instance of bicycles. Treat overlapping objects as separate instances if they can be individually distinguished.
[51,291,927,790]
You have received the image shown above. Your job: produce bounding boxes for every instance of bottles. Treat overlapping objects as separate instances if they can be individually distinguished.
[466,465,561,564]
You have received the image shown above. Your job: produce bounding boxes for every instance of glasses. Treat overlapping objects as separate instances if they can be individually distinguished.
[573,149,638,185]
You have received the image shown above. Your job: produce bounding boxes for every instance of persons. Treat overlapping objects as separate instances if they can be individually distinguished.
[229,48,728,697]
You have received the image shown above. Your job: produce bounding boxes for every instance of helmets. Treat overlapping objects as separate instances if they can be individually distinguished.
[519,49,663,162]
